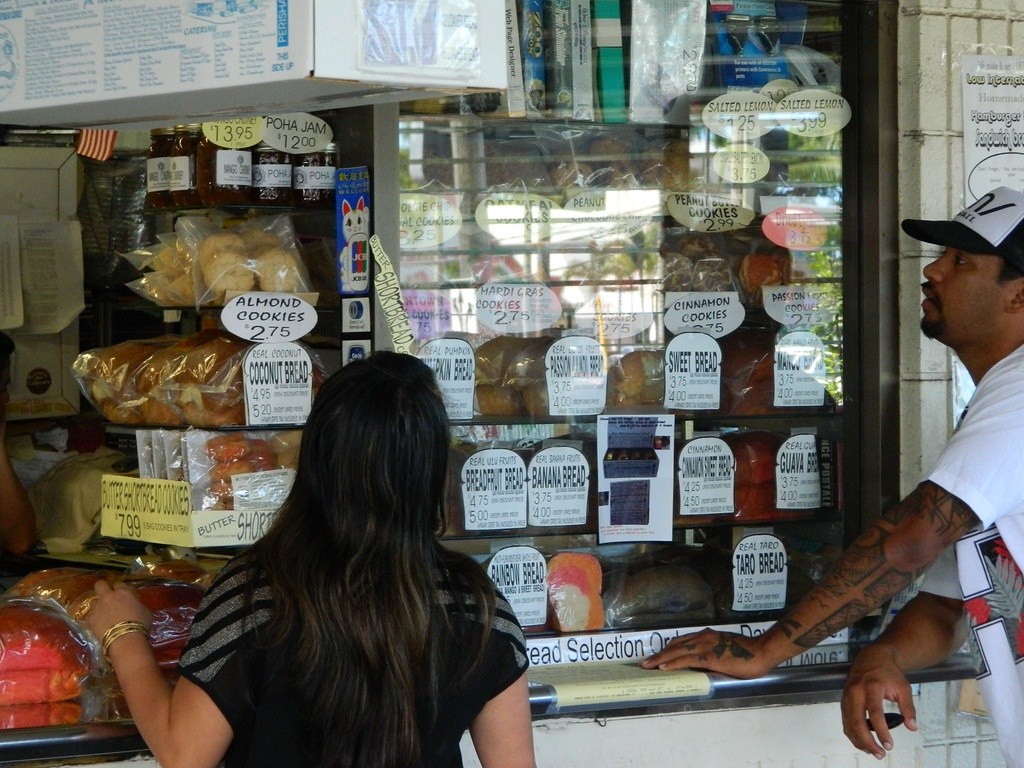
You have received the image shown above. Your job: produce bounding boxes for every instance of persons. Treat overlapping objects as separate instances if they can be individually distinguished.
[640,185,1024,768]
[0,331,37,591]
[70,350,536,768]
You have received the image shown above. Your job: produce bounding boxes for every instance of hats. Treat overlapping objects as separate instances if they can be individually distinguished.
[901,186,1024,261]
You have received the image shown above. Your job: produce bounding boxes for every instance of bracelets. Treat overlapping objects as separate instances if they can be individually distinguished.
[102,619,150,666]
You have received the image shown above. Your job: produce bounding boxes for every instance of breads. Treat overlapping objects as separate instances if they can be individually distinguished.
[0,558,228,730]
[80,218,306,513]
[439,138,840,629]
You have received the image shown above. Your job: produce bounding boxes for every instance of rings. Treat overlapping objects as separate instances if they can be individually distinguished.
[74,595,99,622]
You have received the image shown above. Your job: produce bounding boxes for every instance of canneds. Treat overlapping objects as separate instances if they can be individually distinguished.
[147,122,338,207]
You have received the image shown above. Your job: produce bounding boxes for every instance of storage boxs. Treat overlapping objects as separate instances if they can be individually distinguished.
[0,0,507,129]
